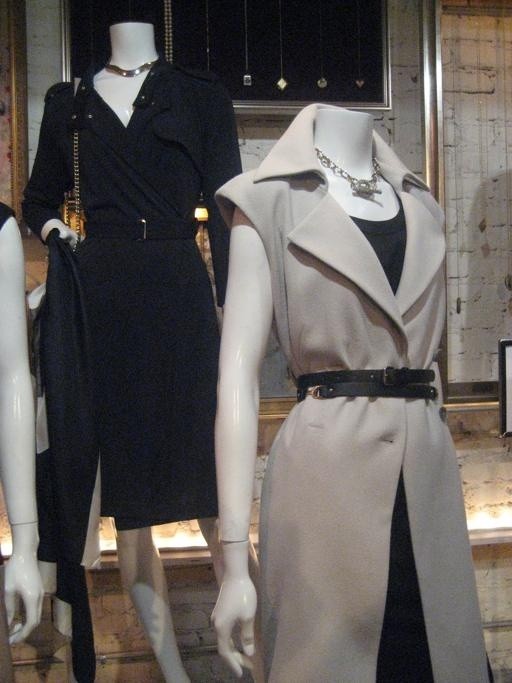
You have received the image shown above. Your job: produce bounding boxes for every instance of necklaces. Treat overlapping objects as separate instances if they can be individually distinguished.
[100,61,156,77]
[316,144,387,197]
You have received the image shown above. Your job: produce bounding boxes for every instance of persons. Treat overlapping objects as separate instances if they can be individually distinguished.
[21,21,259,681]
[208,100,498,683]
[0,198,46,682]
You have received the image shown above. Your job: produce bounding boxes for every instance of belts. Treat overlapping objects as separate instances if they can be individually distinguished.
[297,365,439,402]
[83,218,199,242]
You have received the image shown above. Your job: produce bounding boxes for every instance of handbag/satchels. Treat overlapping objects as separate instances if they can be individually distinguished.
[58,189,85,243]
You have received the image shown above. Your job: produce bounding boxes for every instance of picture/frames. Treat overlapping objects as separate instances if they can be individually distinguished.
[58,0,392,117]
